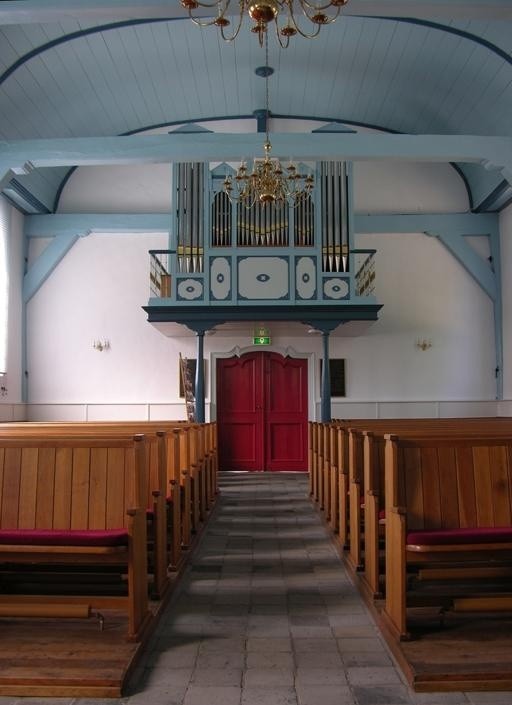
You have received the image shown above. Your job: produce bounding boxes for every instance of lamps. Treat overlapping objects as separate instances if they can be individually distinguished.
[222,26,316,209]
[177,0,353,50]
